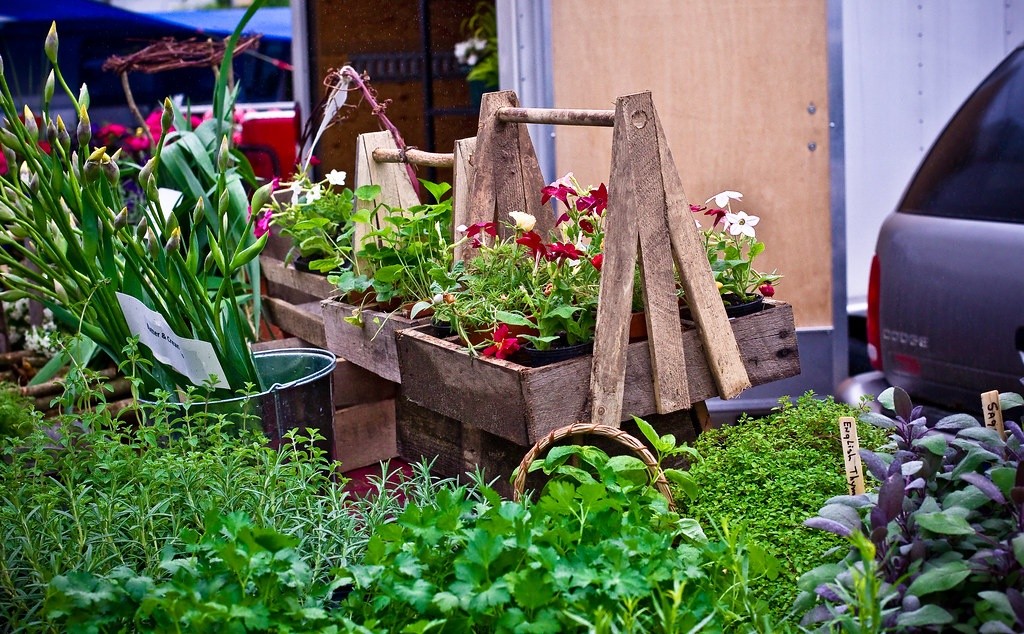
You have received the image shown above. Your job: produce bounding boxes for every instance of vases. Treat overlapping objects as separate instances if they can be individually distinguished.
[134,346,351,603]
[430,291,764,369]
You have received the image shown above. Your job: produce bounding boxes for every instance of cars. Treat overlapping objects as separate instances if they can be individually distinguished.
[834,41,1024,433]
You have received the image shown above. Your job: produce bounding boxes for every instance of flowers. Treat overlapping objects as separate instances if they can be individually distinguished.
[0,0,280,406]
[432,172,786,359]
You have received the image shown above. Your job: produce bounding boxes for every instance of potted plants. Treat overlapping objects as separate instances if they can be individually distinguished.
[269,177,452,321]
